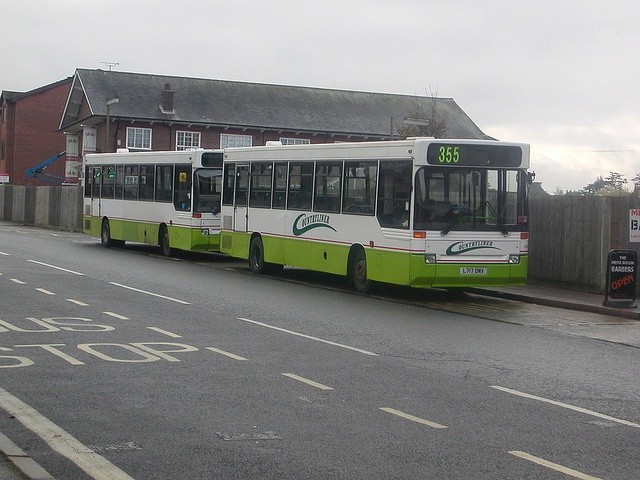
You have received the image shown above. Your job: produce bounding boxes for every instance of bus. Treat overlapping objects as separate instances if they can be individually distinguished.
[81,149,225,256]
[220,138,535,292]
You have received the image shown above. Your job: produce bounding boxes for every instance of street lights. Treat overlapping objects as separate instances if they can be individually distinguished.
[105,96,119,153]
